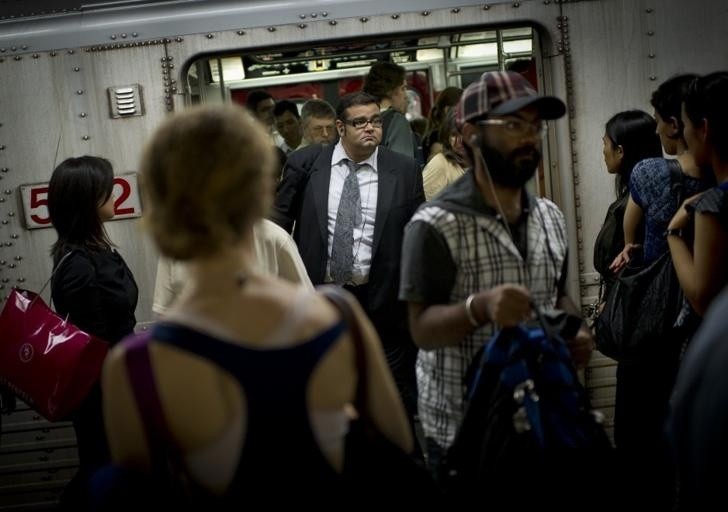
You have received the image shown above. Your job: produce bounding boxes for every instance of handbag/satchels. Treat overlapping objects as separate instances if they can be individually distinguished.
[611,158,699,340]
[2,249,113,422]
[673,288,704,365]
[314,284,437,511]
[591,158,683,361]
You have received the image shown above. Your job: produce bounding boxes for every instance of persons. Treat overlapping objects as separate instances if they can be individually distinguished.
[268,90,429,315]
[592,109,663,450]
[246,90,276,127]
[102,103,432,511]
[361,62,414,161]
[399,68,594,512]
[421,87,465,165]
[272,101,303,156]
[421,106,474,203]
[608,73,705,446]
[151,215,318,323]
[278,99,337,182]
[43,153,141,472]
[664,69,728,377]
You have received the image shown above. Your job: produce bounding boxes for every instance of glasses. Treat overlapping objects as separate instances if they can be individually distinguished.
[480,118,547,142]
[342,117,385,129]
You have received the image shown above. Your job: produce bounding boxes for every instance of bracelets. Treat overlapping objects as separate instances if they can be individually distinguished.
[465,292,480,328]
[662,227,688,239]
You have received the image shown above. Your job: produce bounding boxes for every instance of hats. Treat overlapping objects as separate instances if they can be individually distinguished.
[405,90,425,122]
[455,71,565,130]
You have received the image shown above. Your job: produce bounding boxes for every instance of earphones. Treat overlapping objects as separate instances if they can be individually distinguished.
[467,134,512,237]
[339,128,376,265]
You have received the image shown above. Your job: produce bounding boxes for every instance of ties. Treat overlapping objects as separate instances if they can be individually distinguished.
[330,160,363,290]
